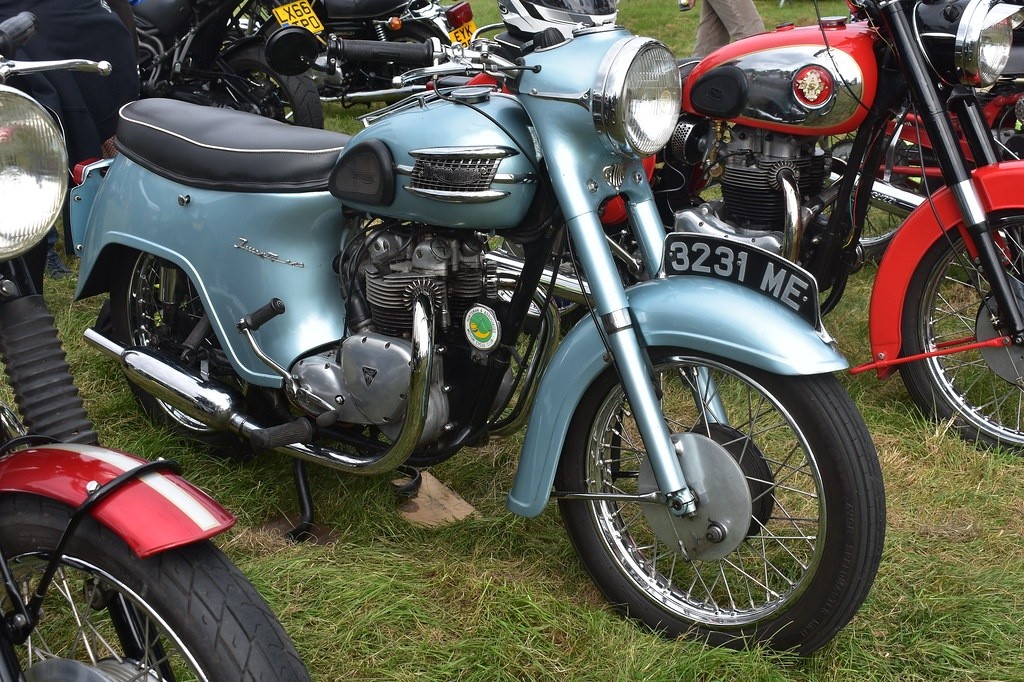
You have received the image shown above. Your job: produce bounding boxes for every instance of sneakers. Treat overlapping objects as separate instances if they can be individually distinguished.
[45,247,68,279]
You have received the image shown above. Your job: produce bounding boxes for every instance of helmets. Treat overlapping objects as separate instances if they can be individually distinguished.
[495,0,618,42]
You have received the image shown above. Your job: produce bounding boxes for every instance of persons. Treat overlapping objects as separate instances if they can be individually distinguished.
[0,0,140,189]
[688,0,765,59]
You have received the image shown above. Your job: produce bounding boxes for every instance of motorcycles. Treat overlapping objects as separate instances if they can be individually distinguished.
[0,0,1024,660]
[0,9,313,682]
